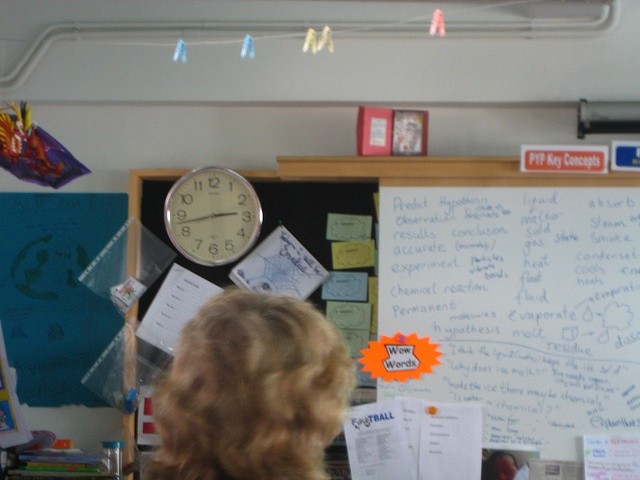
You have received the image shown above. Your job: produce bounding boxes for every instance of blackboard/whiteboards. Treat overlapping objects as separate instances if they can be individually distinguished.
[137,180,376,389]
[377,186,640,461]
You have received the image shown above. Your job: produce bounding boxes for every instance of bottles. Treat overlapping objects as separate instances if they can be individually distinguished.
[101,440,124,478]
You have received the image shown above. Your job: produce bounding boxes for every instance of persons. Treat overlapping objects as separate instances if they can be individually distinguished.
[141,287,355,480]
[483,451,519,479]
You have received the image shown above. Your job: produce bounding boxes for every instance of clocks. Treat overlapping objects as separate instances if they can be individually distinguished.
[163,165,263,267]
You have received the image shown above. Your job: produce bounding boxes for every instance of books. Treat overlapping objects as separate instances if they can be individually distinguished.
[6,455,109,476]
[227,222,332,303]
